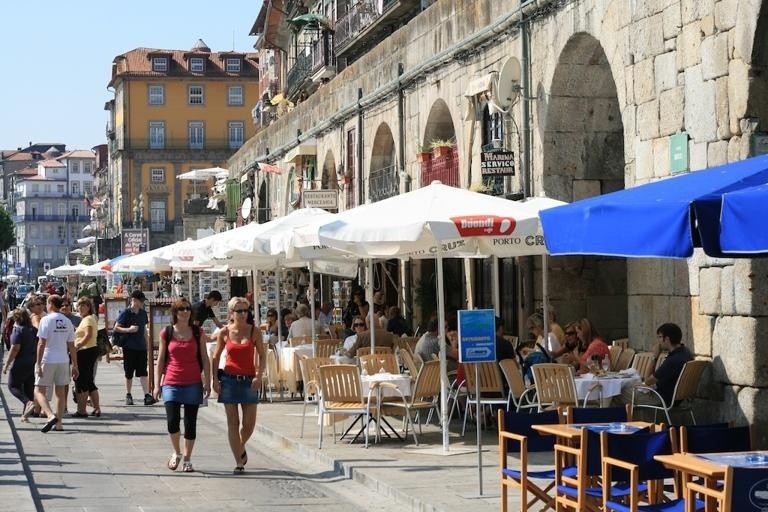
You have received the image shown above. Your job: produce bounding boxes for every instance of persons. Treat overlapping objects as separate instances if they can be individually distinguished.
[339,292,412,360]
[212,296,266,475]
[480,316,611,400]
[153,297,212,473]
[414,316,458,360]
[0,271,160,434]
[267,286,334,344]
[611,321,694,408]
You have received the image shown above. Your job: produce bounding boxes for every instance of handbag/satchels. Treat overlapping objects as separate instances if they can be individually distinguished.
[111,330,129,347]
[522,352,557,384]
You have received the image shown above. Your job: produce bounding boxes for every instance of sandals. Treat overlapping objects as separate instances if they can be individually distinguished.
[233,450,247,473]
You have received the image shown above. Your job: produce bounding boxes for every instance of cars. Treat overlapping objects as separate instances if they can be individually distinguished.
[13,279,64,308]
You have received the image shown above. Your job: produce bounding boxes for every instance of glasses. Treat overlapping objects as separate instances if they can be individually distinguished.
[177,306,192,311]
[234,309,247,313]
[267,314,275,317]
[353,323,365,327]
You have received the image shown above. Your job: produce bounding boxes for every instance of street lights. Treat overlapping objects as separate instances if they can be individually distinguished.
[132,197,138,229]
[139,192,146,252]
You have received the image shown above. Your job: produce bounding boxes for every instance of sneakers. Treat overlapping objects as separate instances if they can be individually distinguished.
[20,385,101,432]
[143,395,156,404]
[126,395,133,405]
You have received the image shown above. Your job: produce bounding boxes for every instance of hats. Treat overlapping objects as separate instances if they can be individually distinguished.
[130,290,149,301]
[295,304,308,317]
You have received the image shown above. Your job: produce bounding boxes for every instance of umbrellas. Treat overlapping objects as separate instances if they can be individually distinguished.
[438,196,573,320]
[292,179,545,449]
[536,153,768,262]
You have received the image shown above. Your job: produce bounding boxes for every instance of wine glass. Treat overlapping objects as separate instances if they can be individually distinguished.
[361,359,387,380]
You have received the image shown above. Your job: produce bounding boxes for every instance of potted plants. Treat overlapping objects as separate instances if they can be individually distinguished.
[340,168,352,184]
[415,136,453,165]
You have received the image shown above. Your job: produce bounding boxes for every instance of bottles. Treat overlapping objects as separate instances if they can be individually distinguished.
[338,341,344,355]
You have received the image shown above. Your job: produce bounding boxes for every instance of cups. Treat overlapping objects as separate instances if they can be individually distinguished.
[588,353,610,380]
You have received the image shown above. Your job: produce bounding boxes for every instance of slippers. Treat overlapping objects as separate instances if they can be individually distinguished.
[167,452,182,470]
[183,461,192,472]
